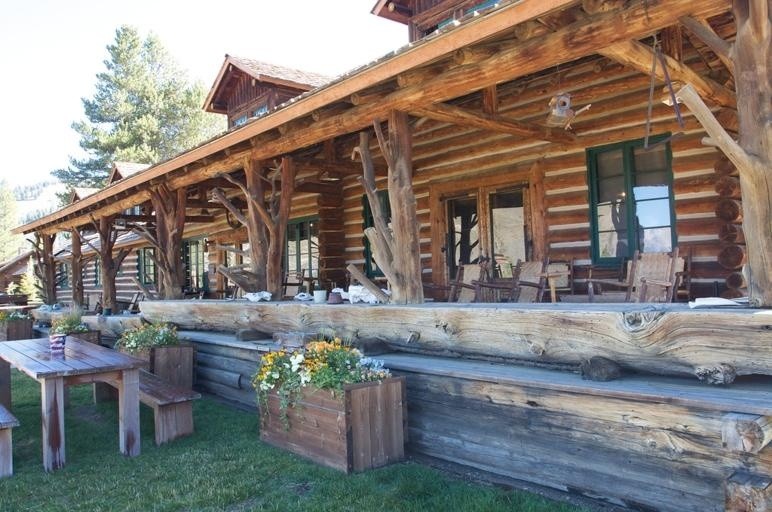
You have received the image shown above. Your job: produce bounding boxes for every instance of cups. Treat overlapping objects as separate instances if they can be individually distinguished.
[103,308,112,316]
[327,292,344,304]
[313,290,327,304]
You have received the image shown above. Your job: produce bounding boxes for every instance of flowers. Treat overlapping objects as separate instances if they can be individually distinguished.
[114,317,179,355]
[48,301,91,334]
[0,310,35,321]
[249,326,392,432]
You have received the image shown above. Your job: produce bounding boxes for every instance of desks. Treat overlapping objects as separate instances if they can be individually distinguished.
[0,335,147,474]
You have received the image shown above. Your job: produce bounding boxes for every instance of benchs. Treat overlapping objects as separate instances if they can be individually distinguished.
[93,368,202,446]
[0,403,20,476]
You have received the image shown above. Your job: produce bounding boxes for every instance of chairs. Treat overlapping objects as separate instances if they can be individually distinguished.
[422,247,693,302]
[280,266,355,301]
